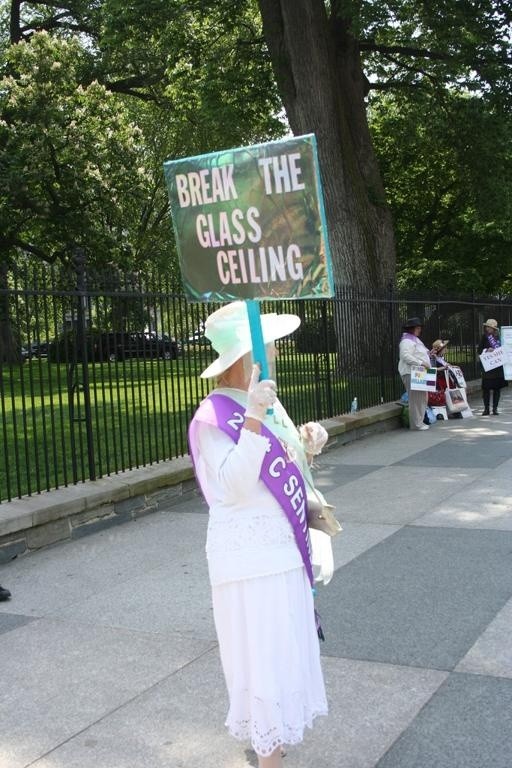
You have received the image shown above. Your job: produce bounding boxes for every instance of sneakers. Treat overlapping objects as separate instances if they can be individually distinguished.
[417,424,430,430]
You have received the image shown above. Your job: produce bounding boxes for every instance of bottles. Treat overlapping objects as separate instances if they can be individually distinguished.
[351,397,358,413]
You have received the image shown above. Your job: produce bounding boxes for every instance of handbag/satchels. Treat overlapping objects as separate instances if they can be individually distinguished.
[305,498,343,537]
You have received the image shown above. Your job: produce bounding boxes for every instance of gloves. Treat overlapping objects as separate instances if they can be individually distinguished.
[299,421,329,455]
[244,364,277,423]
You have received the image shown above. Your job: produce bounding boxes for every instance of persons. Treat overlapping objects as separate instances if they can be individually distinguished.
[0,586,12,600]
[427,339,462,419]
[397,317,438,431]
[188,300,331,768]
[477,318,508,416]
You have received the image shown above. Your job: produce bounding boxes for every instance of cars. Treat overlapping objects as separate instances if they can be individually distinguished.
[20,309,211,362]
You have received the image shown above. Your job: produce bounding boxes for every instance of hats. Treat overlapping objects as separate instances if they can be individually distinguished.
[433,339,449,353]
[200,300,301,378]
[483,318,499,330]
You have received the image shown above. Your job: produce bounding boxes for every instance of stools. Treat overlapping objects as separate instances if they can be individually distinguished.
[431,406,449,422]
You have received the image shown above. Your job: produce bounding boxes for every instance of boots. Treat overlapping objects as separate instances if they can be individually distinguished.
[482,406,489,415]
[493,406,499,416]
